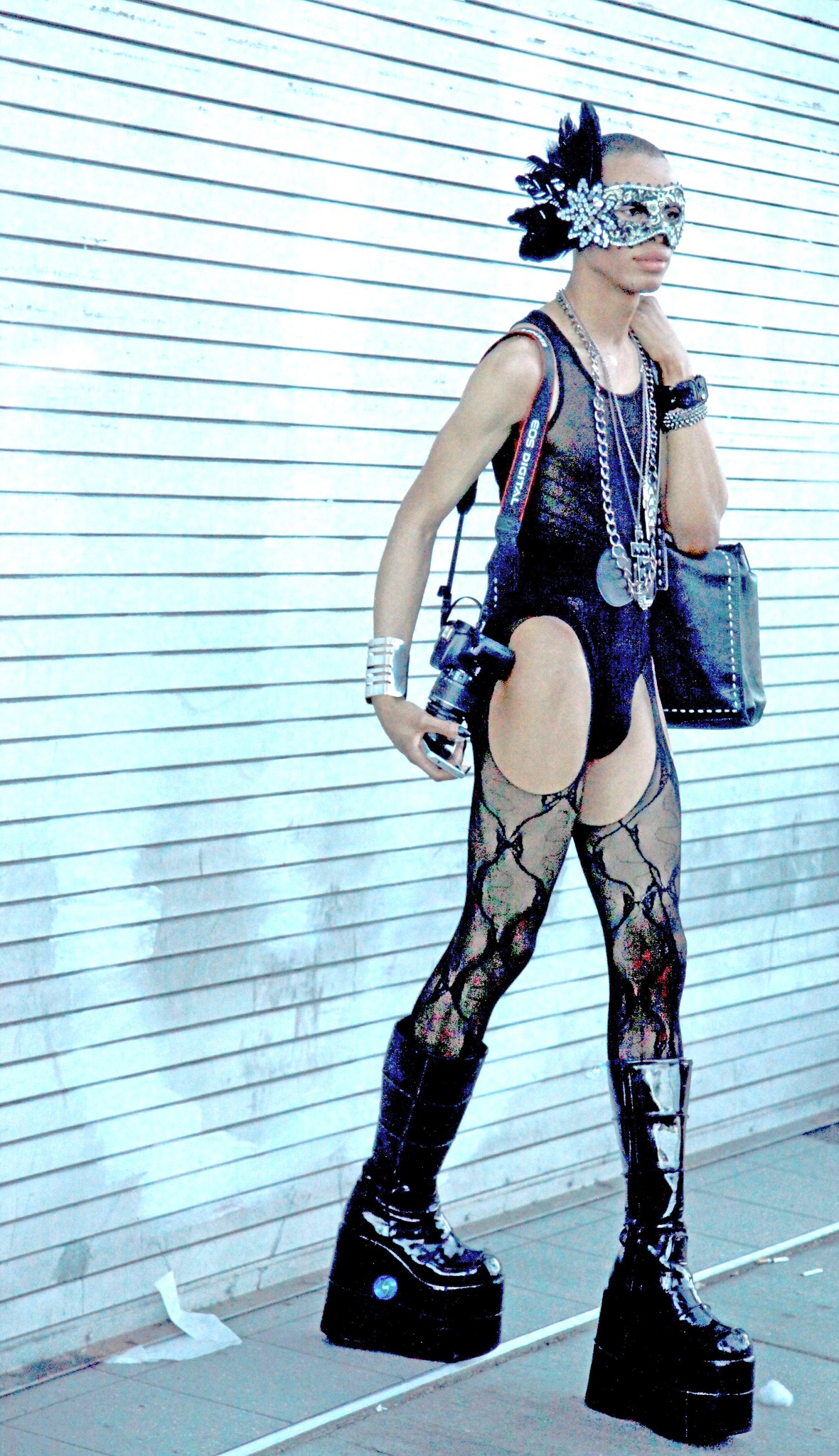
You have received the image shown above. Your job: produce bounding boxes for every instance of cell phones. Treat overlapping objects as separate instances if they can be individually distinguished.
[420,741,466,779]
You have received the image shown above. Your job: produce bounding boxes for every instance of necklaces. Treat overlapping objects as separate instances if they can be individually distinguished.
[554,290,658,611]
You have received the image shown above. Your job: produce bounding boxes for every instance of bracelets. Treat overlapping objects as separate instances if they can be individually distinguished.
[364,637,408,704]
[661,402,709,431]
[660,374,708,409]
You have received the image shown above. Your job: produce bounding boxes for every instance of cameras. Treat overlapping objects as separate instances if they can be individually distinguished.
[424,623,511,745]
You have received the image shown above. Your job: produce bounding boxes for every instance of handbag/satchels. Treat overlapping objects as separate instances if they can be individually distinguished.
[646,530,766,727]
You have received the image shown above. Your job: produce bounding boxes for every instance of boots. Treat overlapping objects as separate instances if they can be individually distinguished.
[327,1017,507,1316]
[591,1056,755,1397]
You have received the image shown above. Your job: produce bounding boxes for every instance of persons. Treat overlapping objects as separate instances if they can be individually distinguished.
[318,99,762,1447]
